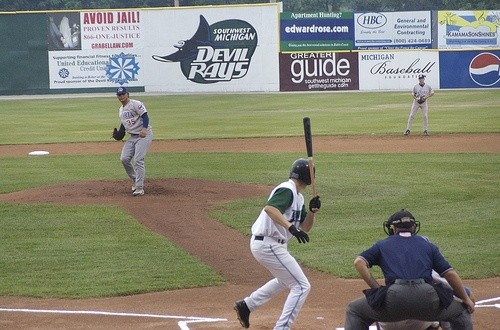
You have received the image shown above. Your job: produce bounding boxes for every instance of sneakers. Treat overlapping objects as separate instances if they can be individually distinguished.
[233,300,250,329]
[132,188,144,196]
[132,186,136,191]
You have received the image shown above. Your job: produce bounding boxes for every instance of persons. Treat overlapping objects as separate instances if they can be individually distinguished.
[345,209,474,330]
[233,158,321,330]
[112,87,153,196]
[404,75,434,135]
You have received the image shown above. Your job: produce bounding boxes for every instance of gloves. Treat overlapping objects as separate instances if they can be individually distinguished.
[288,225,309,243]
[309,195,321,213]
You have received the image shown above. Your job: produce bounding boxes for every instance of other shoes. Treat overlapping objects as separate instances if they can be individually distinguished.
[424,131,429,136]
[404,130,410,135]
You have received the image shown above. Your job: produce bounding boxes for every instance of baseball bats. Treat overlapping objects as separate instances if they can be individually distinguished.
[303,117,318,211]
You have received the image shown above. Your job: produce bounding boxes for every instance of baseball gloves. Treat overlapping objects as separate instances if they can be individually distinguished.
[417,95,426,104]
[113,128,126,141]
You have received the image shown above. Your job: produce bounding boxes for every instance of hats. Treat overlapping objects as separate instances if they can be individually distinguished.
[419,75,424,80]
[388,209,415,226]
[117,87,128,96]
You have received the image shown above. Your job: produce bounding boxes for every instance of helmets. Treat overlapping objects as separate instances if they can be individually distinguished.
[288,158,316,185]
